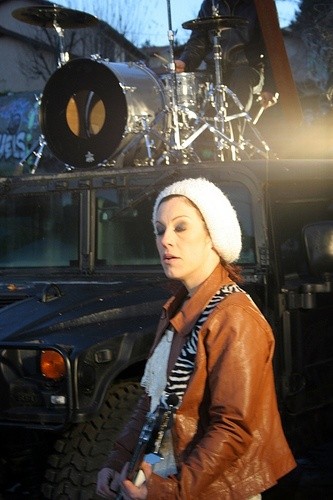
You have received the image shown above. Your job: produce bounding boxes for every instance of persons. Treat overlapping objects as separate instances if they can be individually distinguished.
[172,0,279,160]
[96,179,297,500]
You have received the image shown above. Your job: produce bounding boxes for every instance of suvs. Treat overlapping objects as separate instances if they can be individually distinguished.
[0,152,333,500]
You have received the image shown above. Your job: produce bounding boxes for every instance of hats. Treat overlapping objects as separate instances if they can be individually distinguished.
[152,178,242,263]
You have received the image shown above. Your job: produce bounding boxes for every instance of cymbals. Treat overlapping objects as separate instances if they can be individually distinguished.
[181,15,249,31]
[11,4,99,29]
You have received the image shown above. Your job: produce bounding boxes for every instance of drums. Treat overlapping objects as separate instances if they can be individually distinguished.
[38,57,173,169]
[160,71,203,110]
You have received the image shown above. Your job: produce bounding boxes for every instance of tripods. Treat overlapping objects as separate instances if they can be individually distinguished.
[15,17,71,177]
[114,0,276,170]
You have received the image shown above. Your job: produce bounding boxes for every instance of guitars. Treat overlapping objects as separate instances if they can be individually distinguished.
[113,391,173,500]
